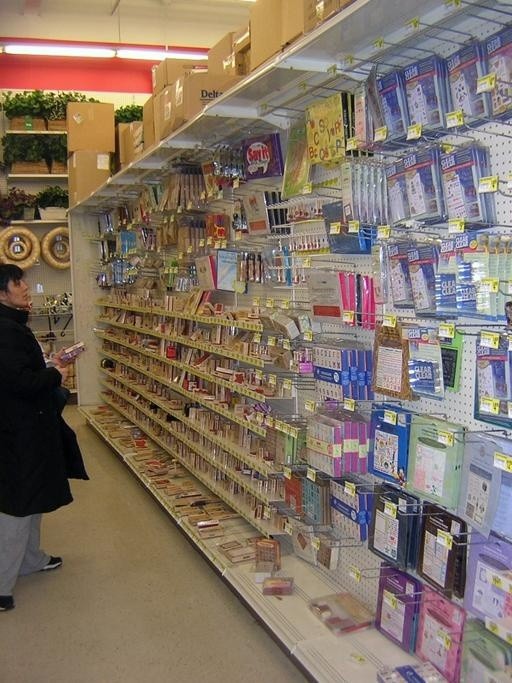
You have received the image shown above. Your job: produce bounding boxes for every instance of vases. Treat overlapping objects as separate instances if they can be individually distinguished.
[23,207,35,220]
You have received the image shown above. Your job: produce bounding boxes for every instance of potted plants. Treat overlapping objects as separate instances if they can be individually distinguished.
[36,184,68,220]
[2,89,49,131]
[47,135,68,174]
[44,90,100,131]
[0,135,49,174]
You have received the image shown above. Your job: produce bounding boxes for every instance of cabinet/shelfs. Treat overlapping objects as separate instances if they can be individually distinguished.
[66,2,512,681]
[0,174,77,395]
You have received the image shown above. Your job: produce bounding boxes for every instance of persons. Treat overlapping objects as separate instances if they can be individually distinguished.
[0,264,90,613]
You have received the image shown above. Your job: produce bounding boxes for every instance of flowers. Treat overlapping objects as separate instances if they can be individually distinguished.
[0,186,36,226]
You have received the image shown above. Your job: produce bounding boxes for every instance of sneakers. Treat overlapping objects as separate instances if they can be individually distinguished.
[0,595,15,611]
[41,556,63,571]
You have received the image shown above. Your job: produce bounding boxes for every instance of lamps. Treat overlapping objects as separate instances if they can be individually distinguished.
[4,43,208,60]
